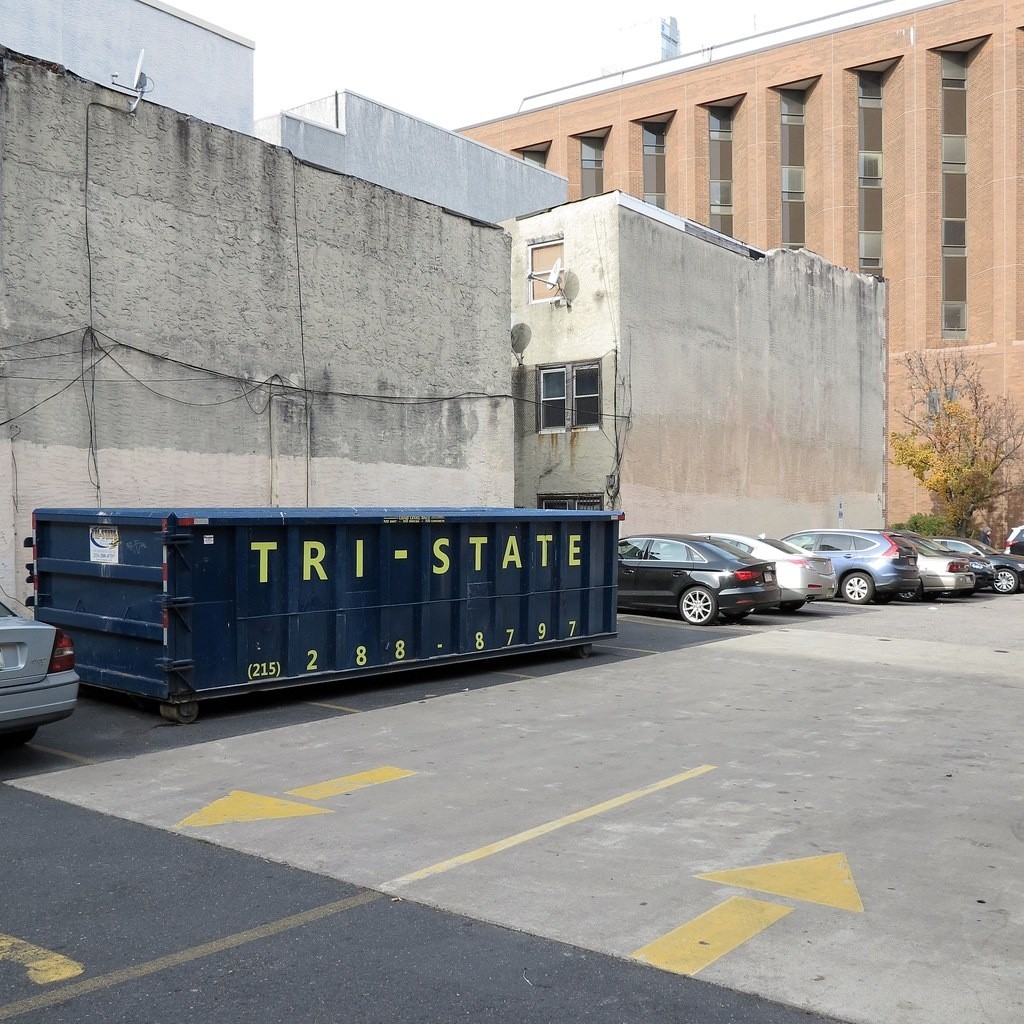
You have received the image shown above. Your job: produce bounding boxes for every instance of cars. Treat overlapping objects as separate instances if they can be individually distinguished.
[0,600,80,746]
[898,541,976,603]
[618,534,783,625]
[898,531,1024,595]
[660,534,837,614]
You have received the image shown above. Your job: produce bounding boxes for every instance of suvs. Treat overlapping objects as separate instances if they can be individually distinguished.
[781,528,920,604]
[1004,526,1024,556]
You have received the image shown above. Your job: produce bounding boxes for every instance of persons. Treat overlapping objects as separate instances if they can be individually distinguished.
[979,526,992,547]
